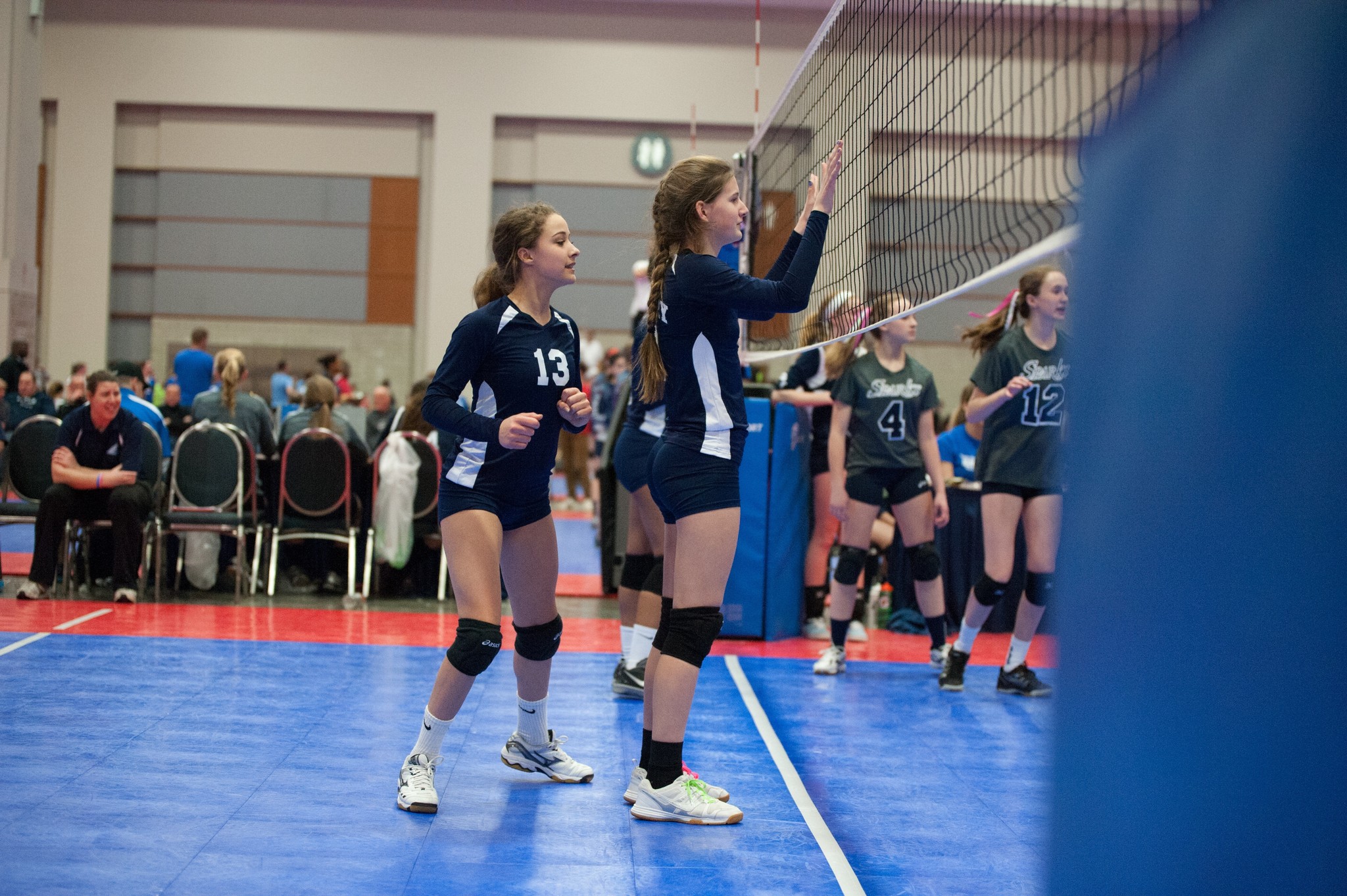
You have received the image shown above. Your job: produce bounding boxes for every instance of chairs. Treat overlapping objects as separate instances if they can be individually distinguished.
[0,414,447,602]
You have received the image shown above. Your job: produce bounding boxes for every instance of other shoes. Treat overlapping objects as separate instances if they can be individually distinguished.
[227,558,264,592]
[16,578,50,599]
[290,569,319,594]
[114,589,137,603]
[847,621,869,642]
[611,657,648,698]
[324,572,347,595]
[801,617,830,640]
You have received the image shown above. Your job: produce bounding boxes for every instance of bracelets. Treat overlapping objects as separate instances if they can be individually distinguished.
[96,471,105,488]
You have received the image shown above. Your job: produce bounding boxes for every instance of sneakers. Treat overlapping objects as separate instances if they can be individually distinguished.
[929,643,967,669]
[500,730,594,785]
[938,649,972,691]
[624,761,731,804]
[997,662,1052,697]
[397,755,444,812]
[814,643,847,675]
[632,778,744,825]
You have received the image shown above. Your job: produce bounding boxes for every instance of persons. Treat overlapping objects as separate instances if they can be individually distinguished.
[394,206,597,813]
[0,265,1071,700]
[622,138,846,819]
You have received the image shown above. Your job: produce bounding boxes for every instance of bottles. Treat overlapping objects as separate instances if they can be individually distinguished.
[868,582,881,629]
[878,582,893,630]
[823,594,832,632]
